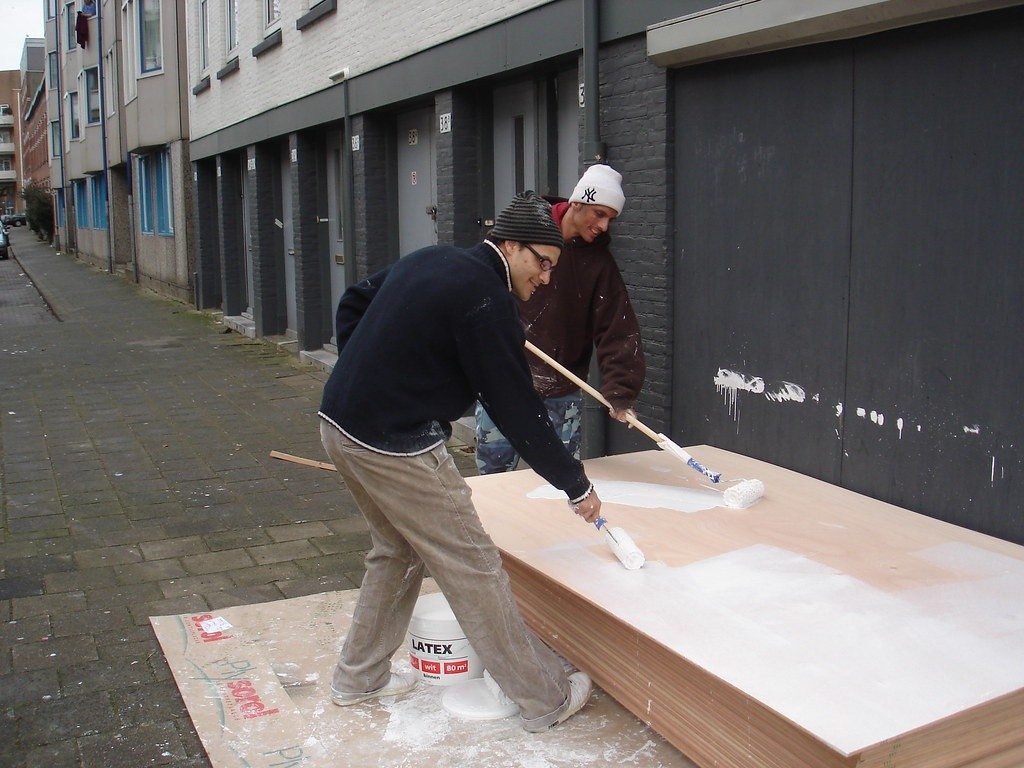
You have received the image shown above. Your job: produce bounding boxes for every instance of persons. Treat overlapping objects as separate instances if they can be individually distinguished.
[317,191,603,733]
[79,0,96,18]
[472,163,646,473]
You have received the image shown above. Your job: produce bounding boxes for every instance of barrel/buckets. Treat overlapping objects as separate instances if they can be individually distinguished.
[406,591,486,686]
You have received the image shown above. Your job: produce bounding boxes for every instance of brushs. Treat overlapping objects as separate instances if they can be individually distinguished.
[656,432,766,508]
[592,517,646,571]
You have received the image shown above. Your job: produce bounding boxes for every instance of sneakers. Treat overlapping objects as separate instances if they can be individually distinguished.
[523,671,592,734]
[330,672,419,706]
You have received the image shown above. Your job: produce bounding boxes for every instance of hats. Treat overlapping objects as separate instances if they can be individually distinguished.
[489,190,565,247]
[568,164,626,218]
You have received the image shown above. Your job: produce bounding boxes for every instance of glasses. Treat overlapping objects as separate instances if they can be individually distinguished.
[522,243,556,274]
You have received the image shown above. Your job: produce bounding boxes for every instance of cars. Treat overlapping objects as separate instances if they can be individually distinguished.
[0,220,11,261]
[4,212,26,227]
